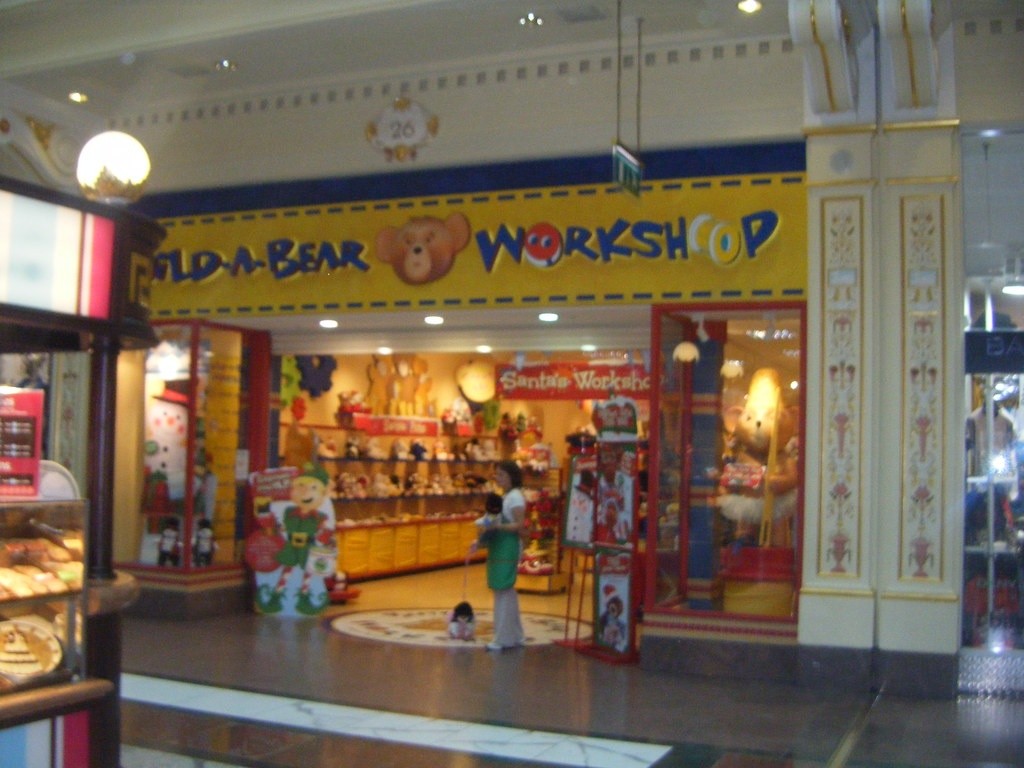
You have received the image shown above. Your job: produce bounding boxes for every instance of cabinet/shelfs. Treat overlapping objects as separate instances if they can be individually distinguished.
[279,420,574,594]
[0,175,167,768]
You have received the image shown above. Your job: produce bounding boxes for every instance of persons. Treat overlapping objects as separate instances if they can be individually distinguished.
[600,596,626,648]
[450,460,524,653]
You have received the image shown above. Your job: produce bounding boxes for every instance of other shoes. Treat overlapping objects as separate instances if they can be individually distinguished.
[486,640,508,650]
